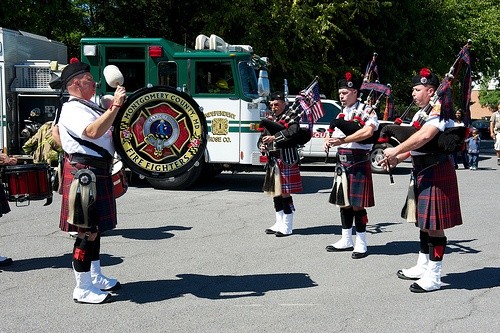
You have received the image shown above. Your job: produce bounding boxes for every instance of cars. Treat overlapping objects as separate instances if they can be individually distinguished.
[281,94,409,174]
[470,119,492,141]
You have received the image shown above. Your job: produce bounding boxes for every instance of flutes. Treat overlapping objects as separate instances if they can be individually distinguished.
[265,72,322,164]
[325,52,392,167]
[379,38,472,184]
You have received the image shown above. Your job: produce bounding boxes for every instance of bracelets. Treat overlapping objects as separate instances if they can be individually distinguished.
[396,158,398,163]
[110,104,121,109]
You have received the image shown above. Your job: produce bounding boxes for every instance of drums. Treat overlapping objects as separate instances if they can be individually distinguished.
[111,83,207,189]
[1,163,53,204]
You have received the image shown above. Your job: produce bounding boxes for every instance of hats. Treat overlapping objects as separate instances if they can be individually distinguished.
[337,73,361,91]
[61,63,91,85]
[269,91,284,101]
[412,68,439,88]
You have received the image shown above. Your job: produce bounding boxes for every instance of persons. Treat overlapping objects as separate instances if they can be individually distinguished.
[52,58,126,304]
[450,108,481,170]
[256,91,302,236]
[378,73,463,293]
[490,102,500,166]
[324,79,380,260]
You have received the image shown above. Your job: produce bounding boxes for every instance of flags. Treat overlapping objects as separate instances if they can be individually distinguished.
[299,81,324,123]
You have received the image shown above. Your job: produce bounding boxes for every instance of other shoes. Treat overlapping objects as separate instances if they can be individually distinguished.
[473,166,478,170]
[469,166,473,170]
[465,165,469,169]
[497,159,500,165]
[0,256,12,265]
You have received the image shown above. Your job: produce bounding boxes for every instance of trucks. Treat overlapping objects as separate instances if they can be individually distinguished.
[0,27,269,186]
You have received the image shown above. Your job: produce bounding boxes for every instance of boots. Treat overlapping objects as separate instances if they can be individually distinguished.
[397,251,430,280]
[71,261,112,304]
[409,259,443,292]
[352,231,367,259]
[274,212,294,236]
[90,260,120,290]
[326,227,353,252]
[266,210,283,234]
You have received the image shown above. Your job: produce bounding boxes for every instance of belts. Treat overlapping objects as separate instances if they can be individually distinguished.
[336,153,371,163]
[68,153,112,170]
[413,154,449,164]
